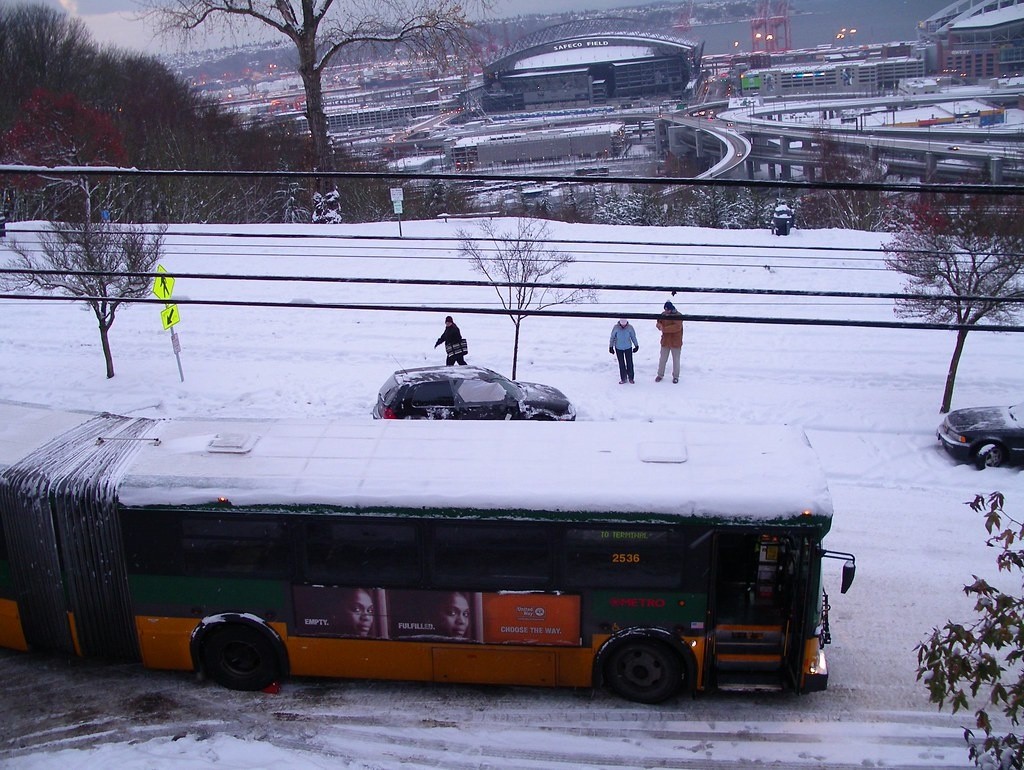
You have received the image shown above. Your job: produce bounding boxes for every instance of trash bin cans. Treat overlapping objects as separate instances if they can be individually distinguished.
[772,206,793,236]
[0,213,6,237]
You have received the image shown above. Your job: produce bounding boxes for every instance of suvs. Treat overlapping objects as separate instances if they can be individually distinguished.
[372,363,578,422]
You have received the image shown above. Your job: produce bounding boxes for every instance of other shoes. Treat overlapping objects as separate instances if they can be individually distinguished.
[619,379,627,384]
[655,376,662,382]
[629,379,635,384]
[673,377,678,383]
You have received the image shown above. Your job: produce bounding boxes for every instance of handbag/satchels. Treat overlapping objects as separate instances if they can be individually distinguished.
[445,338,469,359]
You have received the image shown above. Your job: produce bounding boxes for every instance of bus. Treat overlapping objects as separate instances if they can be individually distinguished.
[1,407,860,706]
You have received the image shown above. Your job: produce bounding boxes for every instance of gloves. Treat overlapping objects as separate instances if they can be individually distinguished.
[633,346,639,353]
[609,346,615,354]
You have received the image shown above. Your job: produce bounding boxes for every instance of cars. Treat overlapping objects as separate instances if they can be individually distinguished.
[936,401,1024,470]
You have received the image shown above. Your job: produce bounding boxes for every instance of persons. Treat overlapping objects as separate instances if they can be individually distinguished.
[341,588,374,636]
[655,301,683,383]
[435,591,469,637]
[609,319,639,384]
[434,316,468,366]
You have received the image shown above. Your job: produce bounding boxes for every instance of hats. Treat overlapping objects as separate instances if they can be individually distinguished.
[444,316,454,322]
[619,318,628,325]
[664,301,673,311]
